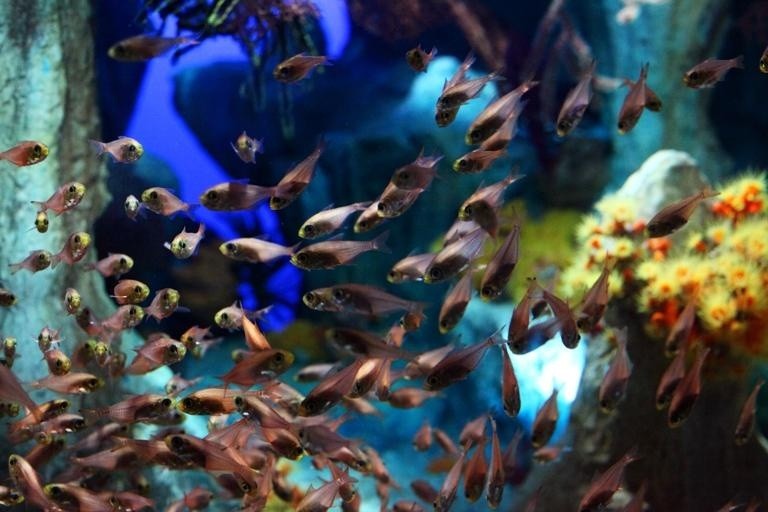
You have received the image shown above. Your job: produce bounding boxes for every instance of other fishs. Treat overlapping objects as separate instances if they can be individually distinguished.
[0,0,768,512]
